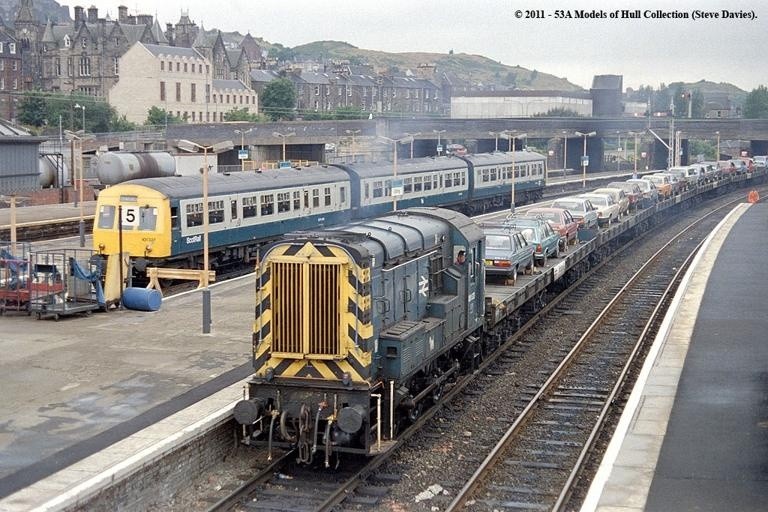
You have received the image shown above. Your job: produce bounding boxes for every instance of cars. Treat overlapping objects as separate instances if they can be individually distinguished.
[483,155,768,285]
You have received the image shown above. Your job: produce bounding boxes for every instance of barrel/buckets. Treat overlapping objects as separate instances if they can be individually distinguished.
[123,286,162,312]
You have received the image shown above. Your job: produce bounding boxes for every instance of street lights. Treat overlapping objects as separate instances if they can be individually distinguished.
[176,137,233,335]
[376,135,414,212]
[432,129,447,157]
[574,130,598,189]
[489,129,529,214]
[233,126,251,170]
[271,131,295,163]
[716,131,719,163]
[628,129,638,179]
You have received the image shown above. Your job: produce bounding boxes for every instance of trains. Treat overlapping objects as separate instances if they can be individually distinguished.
[88,148,548,287]
[232,166,768,468]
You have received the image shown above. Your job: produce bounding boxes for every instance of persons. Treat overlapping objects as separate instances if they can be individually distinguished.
[455,250,465,265]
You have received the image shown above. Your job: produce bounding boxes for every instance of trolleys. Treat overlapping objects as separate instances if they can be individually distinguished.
[0,243,107,321]
